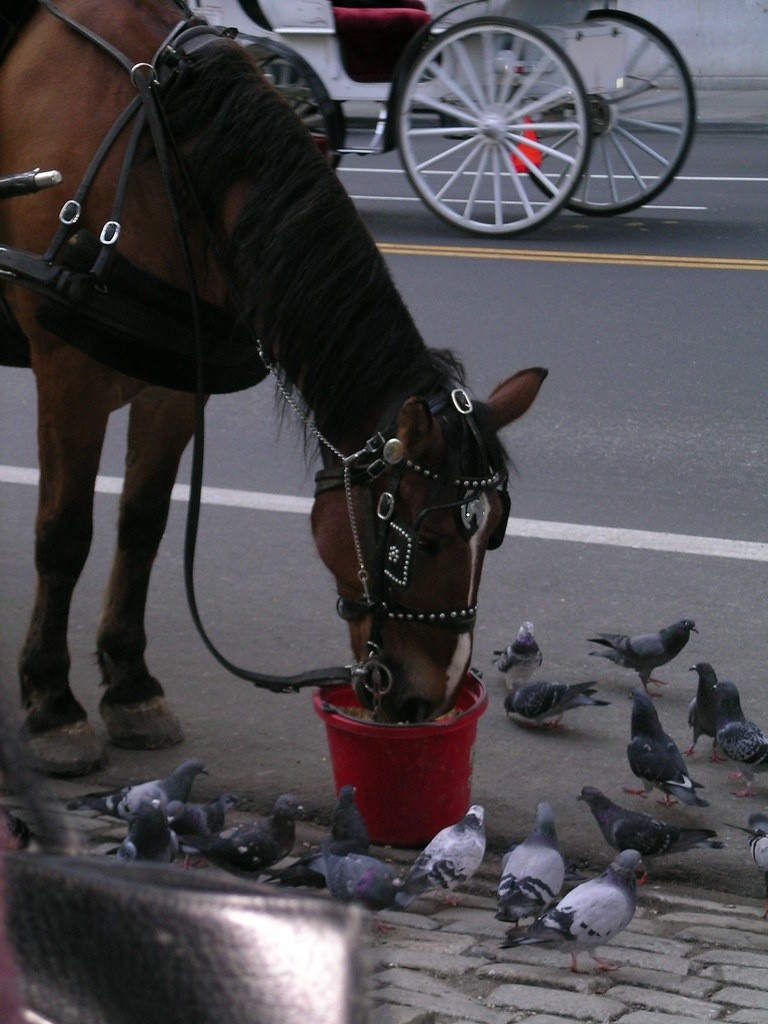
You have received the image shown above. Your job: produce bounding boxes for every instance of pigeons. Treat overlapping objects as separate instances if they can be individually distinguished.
[63,615,768,976]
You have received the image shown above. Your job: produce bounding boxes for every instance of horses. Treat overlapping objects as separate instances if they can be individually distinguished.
[0,0,555,787]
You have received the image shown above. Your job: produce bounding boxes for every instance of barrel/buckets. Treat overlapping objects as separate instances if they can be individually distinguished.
[312,670,488,849]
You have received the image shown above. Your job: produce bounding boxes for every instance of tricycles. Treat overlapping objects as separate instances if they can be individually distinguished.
[191,0,701,239]
